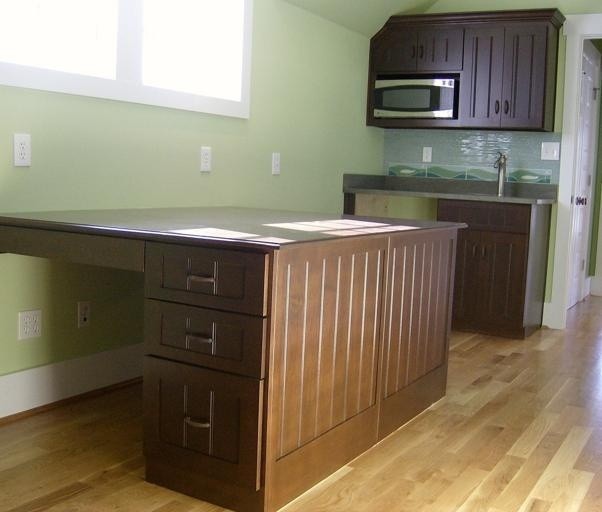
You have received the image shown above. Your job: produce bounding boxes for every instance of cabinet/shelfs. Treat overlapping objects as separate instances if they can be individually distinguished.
[438,199,533,339]
[460,22,558,131]
[371,19,465,74]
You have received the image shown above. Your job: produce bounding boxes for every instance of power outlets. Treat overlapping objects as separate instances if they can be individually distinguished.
[272,152,280,175]
[13,133,31,166]
[77,302,90,329]
[423,147,432,163]
[201,147,211,172]
[18,310,42,338]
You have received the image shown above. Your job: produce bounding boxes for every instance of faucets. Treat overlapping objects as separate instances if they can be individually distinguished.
[494,151,507,197]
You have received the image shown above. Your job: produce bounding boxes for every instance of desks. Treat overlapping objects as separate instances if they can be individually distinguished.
[0,208,470,511]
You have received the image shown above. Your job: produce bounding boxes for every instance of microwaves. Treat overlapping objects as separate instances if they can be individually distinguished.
[372,78,457,120]
